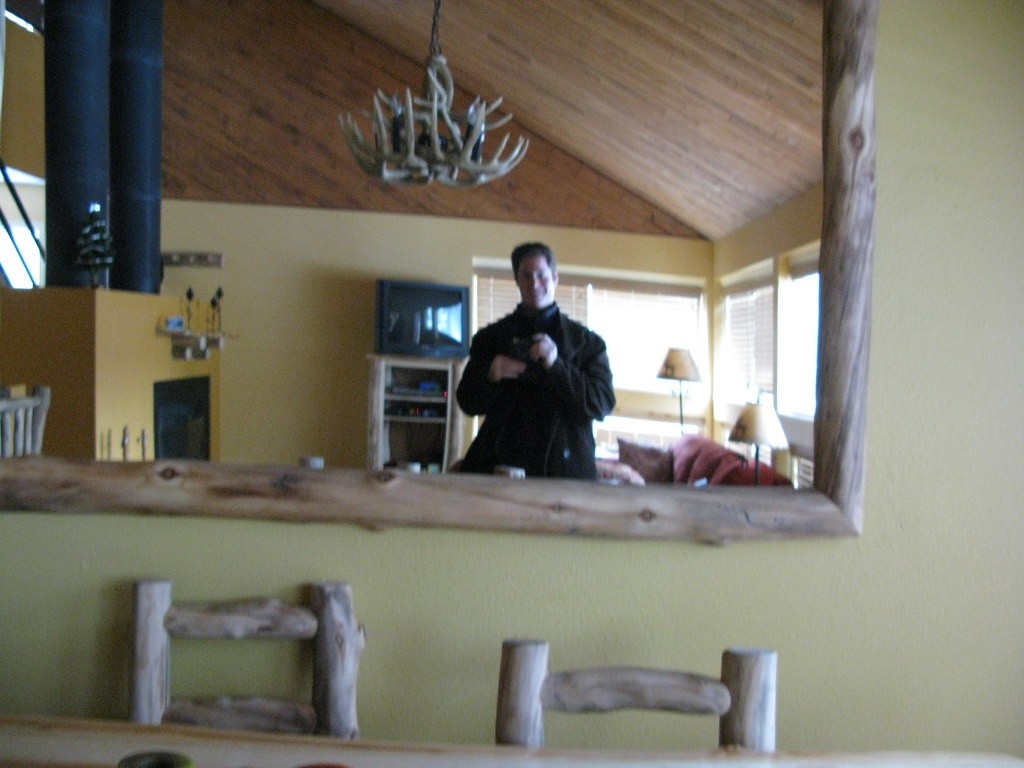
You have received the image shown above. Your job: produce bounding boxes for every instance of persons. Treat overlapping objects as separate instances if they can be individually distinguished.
[454,240,615,484]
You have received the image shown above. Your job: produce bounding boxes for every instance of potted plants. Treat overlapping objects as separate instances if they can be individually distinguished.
[72,201,113,289]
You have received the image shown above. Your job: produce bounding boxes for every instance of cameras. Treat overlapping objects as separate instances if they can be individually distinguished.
[510,338,535,361]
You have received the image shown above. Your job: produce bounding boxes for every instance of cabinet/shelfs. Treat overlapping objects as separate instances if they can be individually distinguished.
[365,353,456,474]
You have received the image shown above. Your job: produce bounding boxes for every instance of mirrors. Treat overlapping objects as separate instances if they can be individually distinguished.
[0,0,879,542]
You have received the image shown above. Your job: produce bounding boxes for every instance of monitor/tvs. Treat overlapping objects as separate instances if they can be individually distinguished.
[376,279,471,358]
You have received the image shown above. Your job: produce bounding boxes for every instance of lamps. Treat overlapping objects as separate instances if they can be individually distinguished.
[728,403,789,486]
[657,348,700,437]
[337,1,530,188]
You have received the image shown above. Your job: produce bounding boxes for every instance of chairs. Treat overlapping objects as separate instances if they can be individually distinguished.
[495,637,777,754]
[129,580,367,742]
